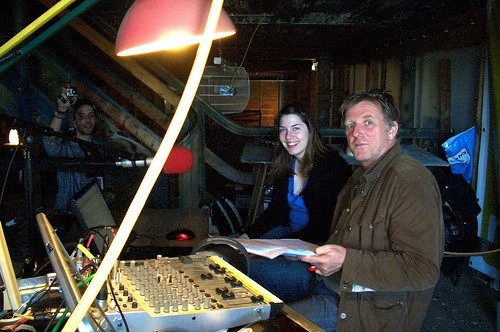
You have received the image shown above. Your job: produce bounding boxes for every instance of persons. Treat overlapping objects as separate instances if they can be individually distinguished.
[44,85,97,245]
[219,106,351,263]
[247,91,445,332]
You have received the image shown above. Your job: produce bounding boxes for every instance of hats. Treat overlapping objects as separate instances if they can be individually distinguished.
[71,96,98,113]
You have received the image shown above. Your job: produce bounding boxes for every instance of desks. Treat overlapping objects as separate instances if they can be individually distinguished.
[0,253,330,332]
[78,207,208,254]
[220,109,262,127]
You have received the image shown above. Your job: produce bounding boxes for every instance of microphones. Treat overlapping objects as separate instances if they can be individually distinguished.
[114,147,194,175]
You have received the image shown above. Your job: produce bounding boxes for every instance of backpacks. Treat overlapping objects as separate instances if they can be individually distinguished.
[436,174,481,279]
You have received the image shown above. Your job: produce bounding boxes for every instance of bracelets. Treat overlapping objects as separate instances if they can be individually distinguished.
[55,110,65,119]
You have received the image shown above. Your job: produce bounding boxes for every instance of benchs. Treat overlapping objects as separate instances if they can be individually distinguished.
[443,236,500,257]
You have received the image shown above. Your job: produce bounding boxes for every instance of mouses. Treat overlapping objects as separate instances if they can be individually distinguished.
[166,229,195,241]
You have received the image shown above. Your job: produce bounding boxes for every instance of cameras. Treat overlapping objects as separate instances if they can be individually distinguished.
[65,88,78,97]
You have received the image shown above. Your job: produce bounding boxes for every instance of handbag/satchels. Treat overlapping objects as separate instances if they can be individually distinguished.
[199,196,243,236]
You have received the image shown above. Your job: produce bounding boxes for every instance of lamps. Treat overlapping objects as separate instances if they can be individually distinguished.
[7,127,22,147]
[113,0,237,58]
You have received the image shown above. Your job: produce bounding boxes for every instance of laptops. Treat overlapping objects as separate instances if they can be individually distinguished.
[70,180,194,261]
